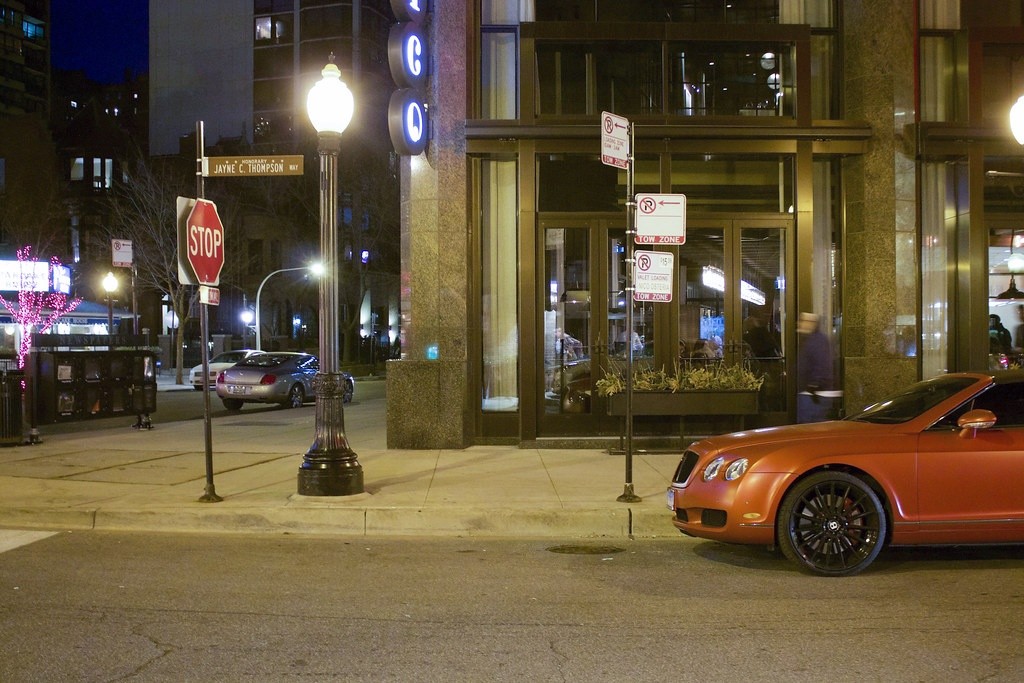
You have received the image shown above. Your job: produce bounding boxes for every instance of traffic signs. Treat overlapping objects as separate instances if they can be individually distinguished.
[601,112,632,171]
[634,192,686,245]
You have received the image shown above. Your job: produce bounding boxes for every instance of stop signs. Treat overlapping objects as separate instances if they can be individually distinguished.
[184,198,225,287]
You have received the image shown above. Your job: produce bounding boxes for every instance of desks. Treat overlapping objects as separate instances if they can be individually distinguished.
[738,108,775,117]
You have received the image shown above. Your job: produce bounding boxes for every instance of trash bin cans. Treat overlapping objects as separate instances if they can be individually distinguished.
[0,352,24,444]
[131,381,157,416]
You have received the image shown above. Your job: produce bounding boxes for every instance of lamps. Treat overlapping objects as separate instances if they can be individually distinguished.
[996,275,1024,300]
[989,228,1024,276]
[702,241,766,306]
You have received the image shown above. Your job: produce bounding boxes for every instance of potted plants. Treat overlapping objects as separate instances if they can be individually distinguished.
[595,358,770,416]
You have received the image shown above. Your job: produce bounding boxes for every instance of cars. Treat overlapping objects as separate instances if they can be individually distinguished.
[214,352,355,410]
[668,363,1024,576]
[188,349,266,390]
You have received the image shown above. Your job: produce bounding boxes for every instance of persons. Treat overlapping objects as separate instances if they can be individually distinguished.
[743,316,780,361]
[130,414,152,428]
[796,313,836,424]
[990,314,1013,352]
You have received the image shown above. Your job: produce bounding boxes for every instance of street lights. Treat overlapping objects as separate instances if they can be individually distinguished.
[288,51,371,498]
[256,265,321,352]
[101,271,119,334]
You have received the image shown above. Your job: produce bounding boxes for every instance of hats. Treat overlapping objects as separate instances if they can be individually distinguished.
[711,336,723,346]
